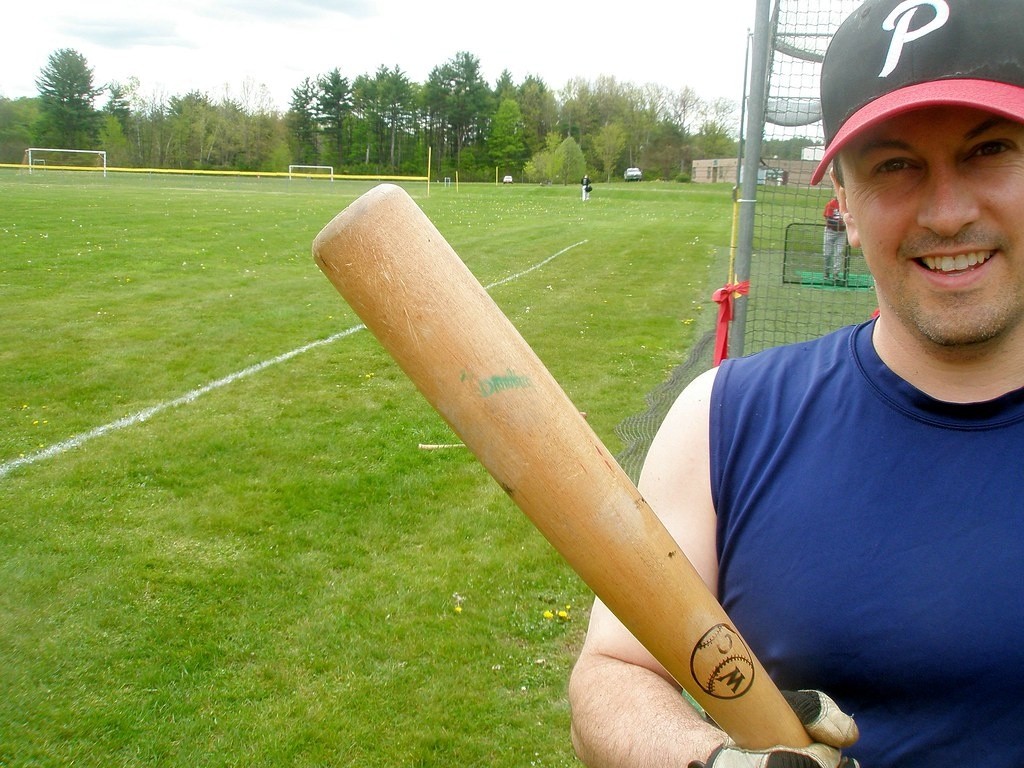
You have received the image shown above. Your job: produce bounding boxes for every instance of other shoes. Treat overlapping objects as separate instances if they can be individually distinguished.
[833,273,840,280]
[824,271,829,279]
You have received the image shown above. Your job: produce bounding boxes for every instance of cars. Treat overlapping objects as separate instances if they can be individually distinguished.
[503,176,513,184]
[624,168,643,182]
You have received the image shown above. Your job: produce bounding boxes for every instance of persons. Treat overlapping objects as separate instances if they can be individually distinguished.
[568,0,1024,768]
[580,175,591,200]
[822,198,846,282]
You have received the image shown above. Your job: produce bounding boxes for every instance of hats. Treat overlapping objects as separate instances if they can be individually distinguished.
[807,0,1023,186]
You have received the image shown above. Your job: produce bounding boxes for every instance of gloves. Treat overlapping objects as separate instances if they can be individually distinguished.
[688,689,859,768]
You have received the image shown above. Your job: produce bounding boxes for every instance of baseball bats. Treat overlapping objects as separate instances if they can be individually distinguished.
[309,181,815,750]
